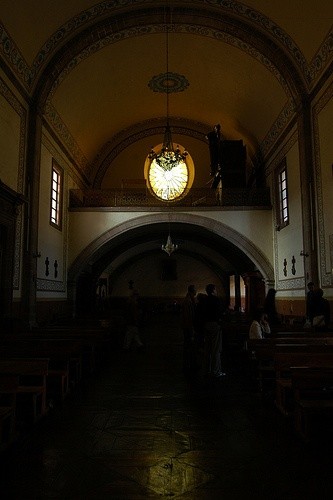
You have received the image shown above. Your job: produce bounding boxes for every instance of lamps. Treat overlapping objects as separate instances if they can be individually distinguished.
[161,223,178,255]
[144,1,188,170]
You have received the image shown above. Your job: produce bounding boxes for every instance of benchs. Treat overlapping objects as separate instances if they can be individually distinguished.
[0,320,111,433]
[235,324,333,438]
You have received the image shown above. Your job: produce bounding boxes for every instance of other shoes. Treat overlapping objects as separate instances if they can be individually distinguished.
[211,371,226,378]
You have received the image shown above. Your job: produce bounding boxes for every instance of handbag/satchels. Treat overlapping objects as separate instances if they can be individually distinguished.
[313,315,325,328]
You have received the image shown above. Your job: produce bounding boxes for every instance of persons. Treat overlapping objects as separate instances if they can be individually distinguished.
[204,124,221,176]
[123,288,143,351]
[181,283,330,378]
